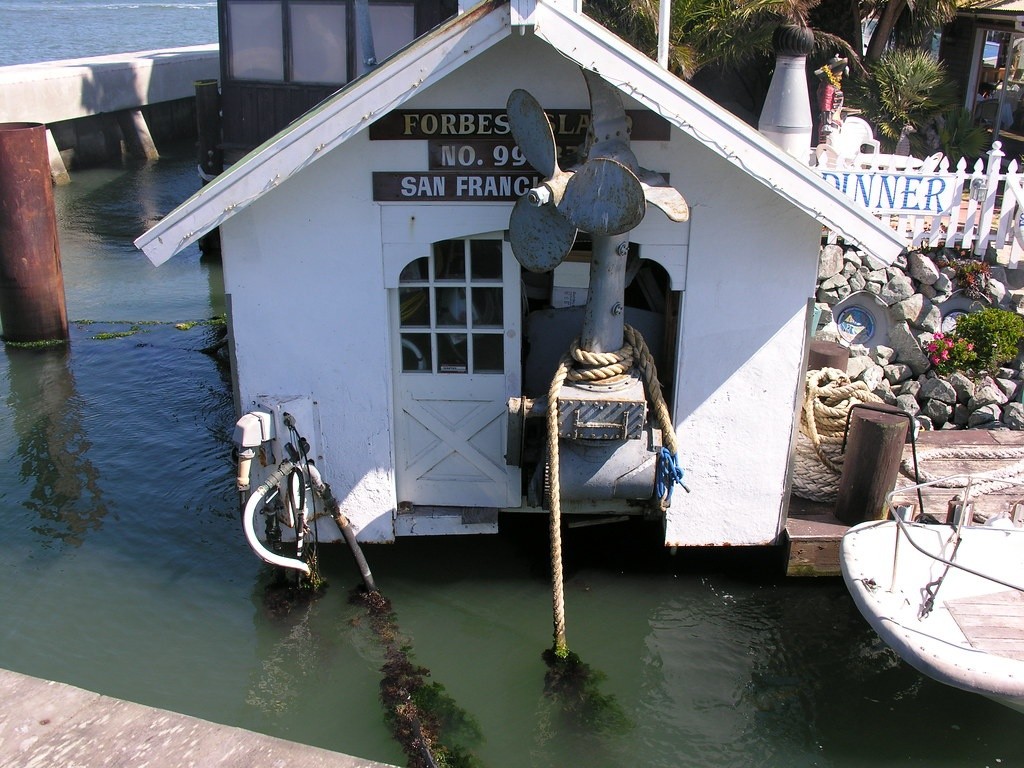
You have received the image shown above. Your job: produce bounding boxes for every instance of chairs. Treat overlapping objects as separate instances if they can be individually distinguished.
[974,99,1000,128]
[816,117,944,231]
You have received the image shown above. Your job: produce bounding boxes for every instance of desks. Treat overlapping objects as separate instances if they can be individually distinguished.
[859,153,923,171]
